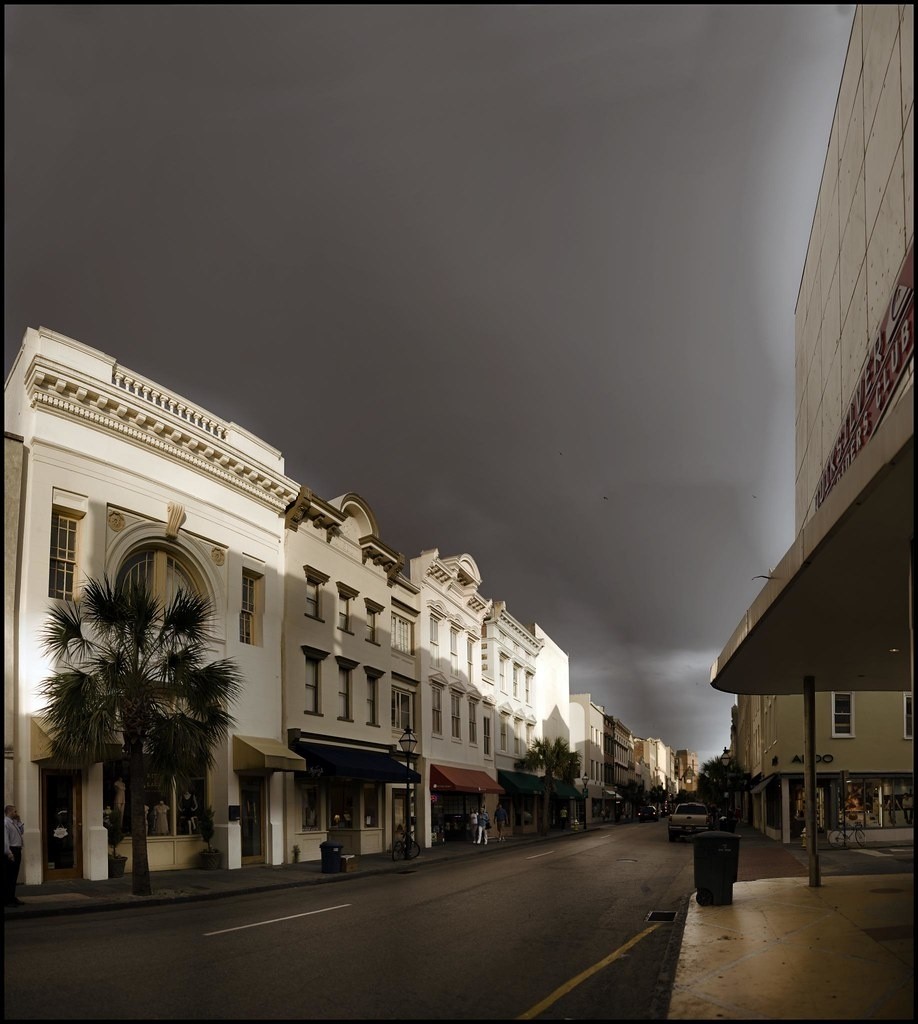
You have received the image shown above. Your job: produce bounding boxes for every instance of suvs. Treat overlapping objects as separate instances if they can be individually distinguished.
[638,806,658,823]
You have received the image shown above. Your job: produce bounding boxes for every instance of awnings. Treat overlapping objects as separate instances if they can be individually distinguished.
[498,769,545,794]
[31,718,123,763]
[296,741,420,784]
[603,790,622,800]
[542,776,581,797]
[430,764,505,794]
[750,776,774,793]
[234,735,306,772]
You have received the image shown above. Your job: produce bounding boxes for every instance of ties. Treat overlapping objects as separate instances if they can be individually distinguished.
[13,822,24,846]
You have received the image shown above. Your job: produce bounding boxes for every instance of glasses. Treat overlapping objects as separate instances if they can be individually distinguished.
[13,811,17,813]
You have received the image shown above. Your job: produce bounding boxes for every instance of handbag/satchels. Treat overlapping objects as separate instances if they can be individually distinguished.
[485,821,492,830]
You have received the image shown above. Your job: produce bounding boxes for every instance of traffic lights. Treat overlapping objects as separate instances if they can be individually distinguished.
[674,762,680,778]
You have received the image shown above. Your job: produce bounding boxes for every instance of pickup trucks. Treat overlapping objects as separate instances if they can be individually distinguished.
[668,801,713,842]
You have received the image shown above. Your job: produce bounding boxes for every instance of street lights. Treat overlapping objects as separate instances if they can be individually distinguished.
[721,747,732,831]
[582,771,589,829]
[398,724,418,860]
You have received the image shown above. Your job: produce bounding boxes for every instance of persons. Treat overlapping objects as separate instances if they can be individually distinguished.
[177,792,198,834]
[847,793,914,824]
[469,807,488,845]
[735,806,742,823]
[4,805,25,907]
[114,778,125,826]
[105,806,113,823]
[712,804,716,821]
[560,807,567,829]
[493,803,508,842]
[600,806,621,822]
[145,801,170,836]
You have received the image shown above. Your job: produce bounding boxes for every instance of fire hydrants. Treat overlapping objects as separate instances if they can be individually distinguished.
[801,827,807,849]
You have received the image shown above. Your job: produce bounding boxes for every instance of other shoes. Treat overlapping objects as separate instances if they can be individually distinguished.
[484,841,488,845]
[502,838,505,842]
[477,841,480,844]
[14,897,25,905]
[497,838,501,842]
[472,840,476,844]
[4,901,17,908]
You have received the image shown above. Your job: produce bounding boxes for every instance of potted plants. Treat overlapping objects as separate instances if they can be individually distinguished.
[108,806,128,879]
[197,805,221,871]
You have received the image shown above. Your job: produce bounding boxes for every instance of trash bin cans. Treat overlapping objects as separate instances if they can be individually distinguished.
[679,831,743,907]
[318,840,344,873]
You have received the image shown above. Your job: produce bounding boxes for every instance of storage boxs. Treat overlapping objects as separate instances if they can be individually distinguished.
[340,857,360,873]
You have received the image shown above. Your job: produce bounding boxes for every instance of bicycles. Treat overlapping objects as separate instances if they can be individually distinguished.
[393,830,420,862]
[828,821,866,848]
